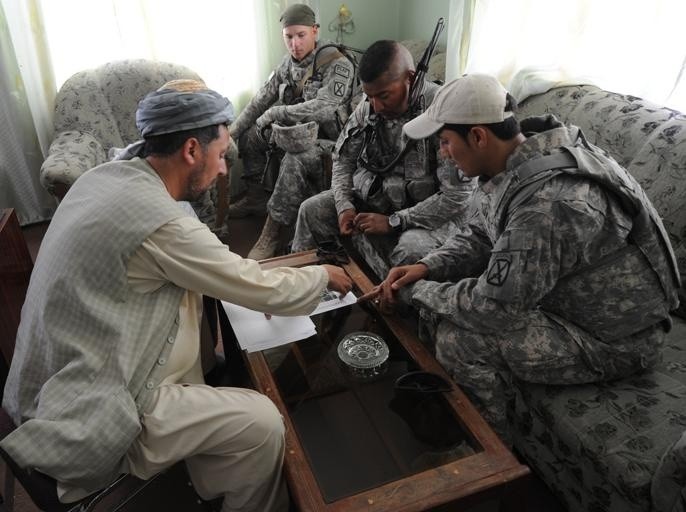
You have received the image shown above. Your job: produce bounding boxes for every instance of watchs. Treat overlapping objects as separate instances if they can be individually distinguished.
[389,212,408,236]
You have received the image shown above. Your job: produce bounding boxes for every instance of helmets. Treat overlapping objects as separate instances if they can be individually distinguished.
[272,120,320,154]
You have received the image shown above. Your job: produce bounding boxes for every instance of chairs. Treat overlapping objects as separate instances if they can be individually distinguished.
[42,62,251,240]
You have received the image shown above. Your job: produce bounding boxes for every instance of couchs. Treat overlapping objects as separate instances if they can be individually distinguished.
[492,66,685,512]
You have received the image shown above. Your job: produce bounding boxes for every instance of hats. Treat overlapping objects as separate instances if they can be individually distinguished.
[401,72,514,139]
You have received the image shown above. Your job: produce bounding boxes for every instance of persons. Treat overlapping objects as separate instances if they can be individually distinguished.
[288,36,485,275]
[227,5,364,261]
[1,86,356,511]
[356,71,683,450]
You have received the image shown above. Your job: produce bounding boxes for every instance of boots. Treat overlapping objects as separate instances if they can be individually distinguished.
[228,183,268,219]
[248,213,285,261]
